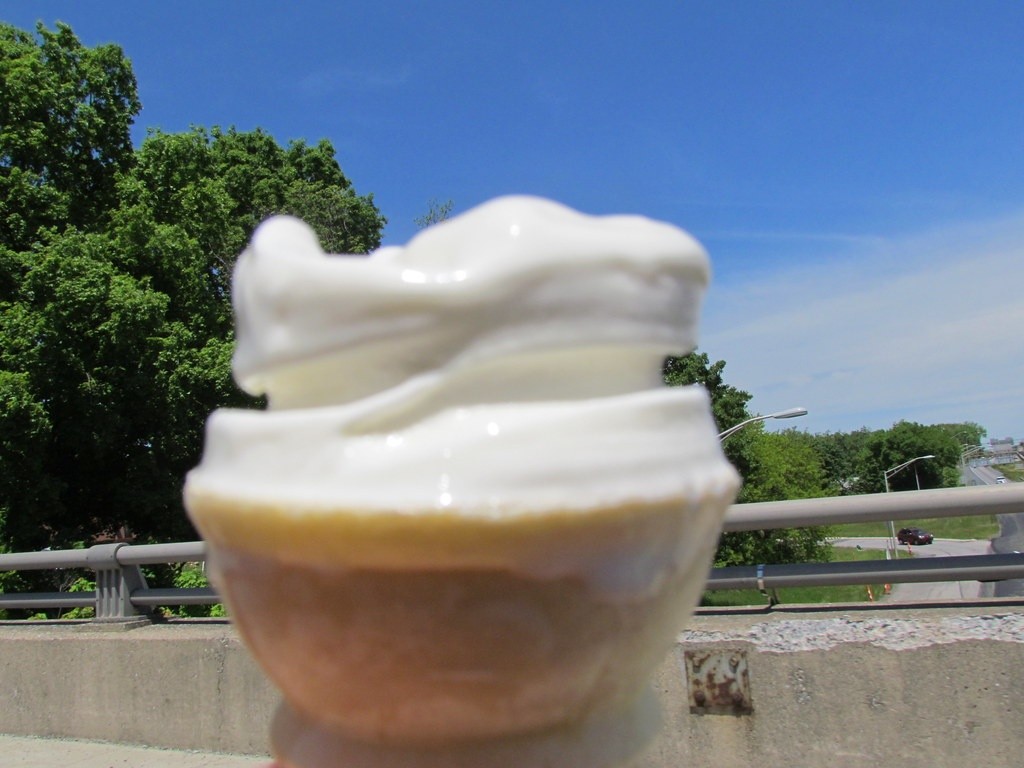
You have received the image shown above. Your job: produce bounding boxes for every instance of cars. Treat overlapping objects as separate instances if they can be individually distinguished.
[996,477,1008,484]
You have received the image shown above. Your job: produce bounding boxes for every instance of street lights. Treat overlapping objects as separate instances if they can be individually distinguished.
[883,454,936,558]
[960,444,985,471]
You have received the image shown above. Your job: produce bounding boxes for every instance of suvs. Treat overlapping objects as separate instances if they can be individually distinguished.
[897,525,934,545]
